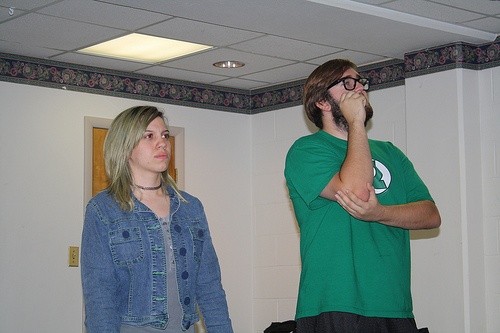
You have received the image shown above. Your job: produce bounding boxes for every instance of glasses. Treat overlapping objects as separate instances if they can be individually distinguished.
[328,78,370,92]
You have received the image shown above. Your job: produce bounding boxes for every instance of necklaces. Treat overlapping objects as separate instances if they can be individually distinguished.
[135,184,161,190]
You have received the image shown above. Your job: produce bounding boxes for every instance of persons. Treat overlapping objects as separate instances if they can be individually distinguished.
[283,58,443,332]
[81,105,234,333]
